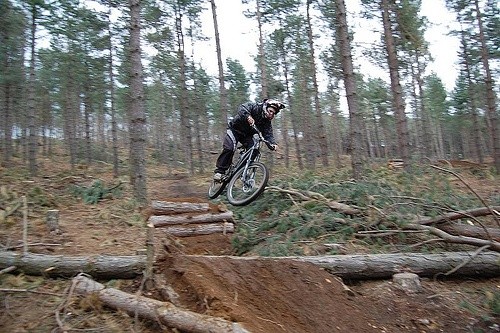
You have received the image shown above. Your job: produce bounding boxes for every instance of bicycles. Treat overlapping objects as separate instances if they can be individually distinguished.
[208,118,277,206]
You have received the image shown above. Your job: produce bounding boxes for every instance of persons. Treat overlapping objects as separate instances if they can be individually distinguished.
[212,98,286,182]
